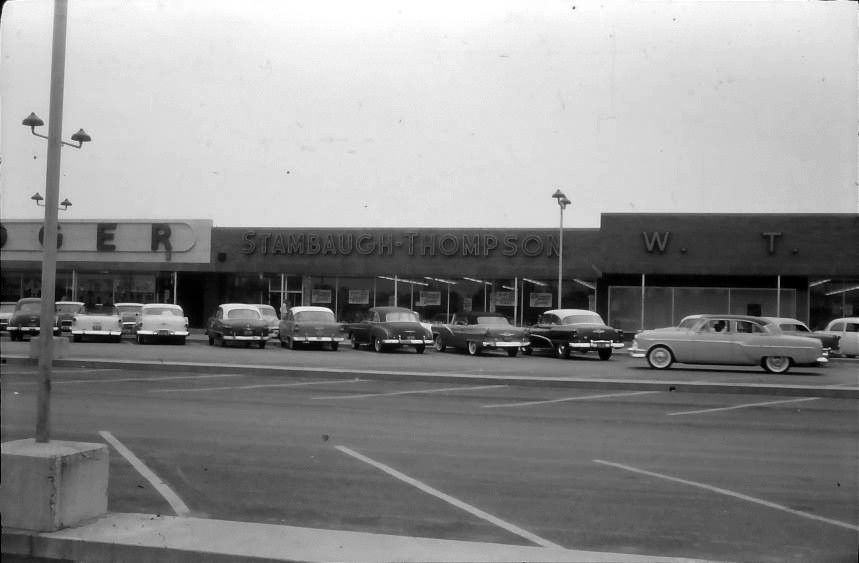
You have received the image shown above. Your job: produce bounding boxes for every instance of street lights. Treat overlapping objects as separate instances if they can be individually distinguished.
[551,189,571,312]
[22,0,94,444]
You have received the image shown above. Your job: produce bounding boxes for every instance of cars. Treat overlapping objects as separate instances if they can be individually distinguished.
[68,299,126,342]
[204,303,271,349]
[432,311,532,355]
[251,303,279,339]
[54,300,82,333]
[0,302,18,335]
[626,312,842,373]
[278,305,345,351]
[6,297,63,341]
[134,302,190,345]
[113,302,144,340]
[521,309,625,360]
[814,317,859,359]
[346,306,435,355]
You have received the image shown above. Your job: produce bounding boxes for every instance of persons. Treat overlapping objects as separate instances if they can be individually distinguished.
[712,320,727,332]
